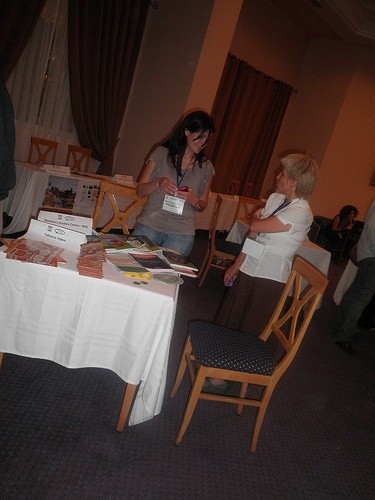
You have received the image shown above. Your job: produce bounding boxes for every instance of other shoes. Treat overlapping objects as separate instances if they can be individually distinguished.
[201,379,229,393]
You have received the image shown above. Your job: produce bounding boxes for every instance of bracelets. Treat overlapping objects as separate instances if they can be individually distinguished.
[194,201,201,210]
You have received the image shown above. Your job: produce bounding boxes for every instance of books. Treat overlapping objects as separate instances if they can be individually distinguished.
[86,233,199,278]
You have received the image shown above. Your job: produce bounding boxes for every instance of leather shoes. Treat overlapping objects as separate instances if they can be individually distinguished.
[335,340,357,356]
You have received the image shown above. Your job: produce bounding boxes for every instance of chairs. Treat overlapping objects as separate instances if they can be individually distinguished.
[226,179,241,196]
[242,182,255,197]
[92,179,149,236]
[232,196,266,227]
[66,144,92,174]
[307,216,340,250]
[171,254,330,453]
[198,194,242,287]
[27,136,58,164]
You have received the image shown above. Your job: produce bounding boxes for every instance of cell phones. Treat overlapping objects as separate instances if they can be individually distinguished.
[178,186,188,191]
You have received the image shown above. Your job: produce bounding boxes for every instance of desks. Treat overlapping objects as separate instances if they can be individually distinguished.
[0,233,181,432]
[3,164,143,234]
[334,233,363,267]
[226,219,331,310]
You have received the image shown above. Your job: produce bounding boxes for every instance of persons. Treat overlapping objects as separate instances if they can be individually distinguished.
[131,110,216,258]
[201,154,320,392]
[323,205,357,262]
[329,199,375,357]
[0,78,17,236]
